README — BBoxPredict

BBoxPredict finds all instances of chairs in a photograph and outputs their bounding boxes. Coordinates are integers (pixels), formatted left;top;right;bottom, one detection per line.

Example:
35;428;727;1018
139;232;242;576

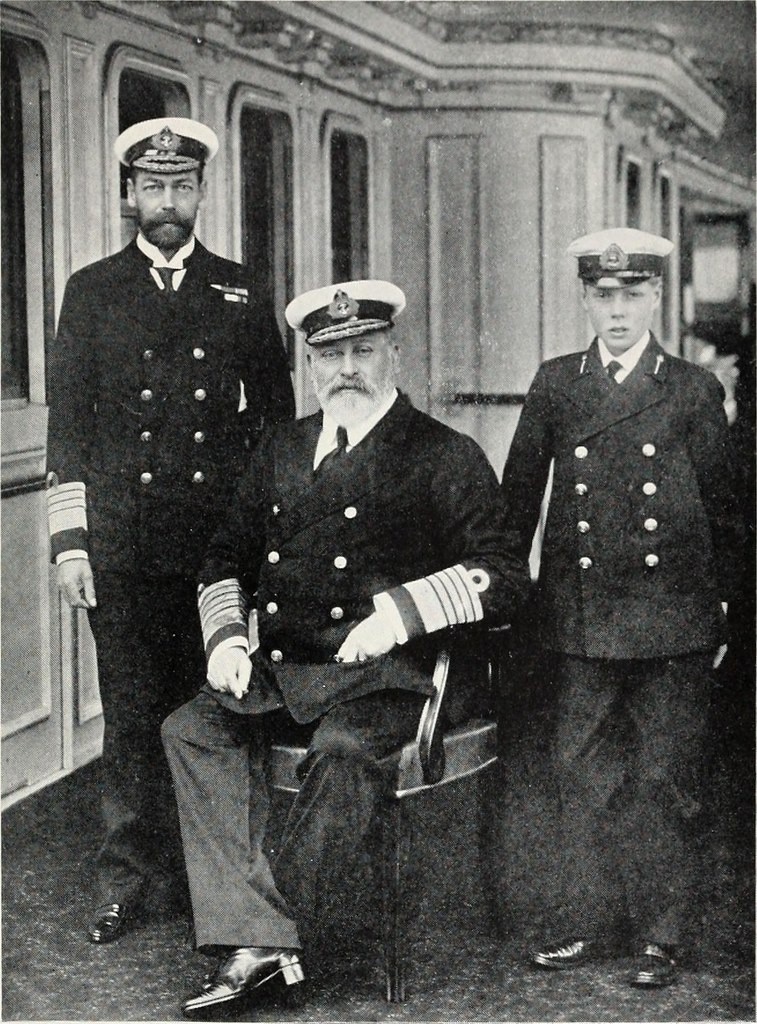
263;619;517;999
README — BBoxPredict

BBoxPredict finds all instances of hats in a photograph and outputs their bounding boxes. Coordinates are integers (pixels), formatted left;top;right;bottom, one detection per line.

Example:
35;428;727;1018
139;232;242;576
566;228;675;289
114;117;220;174
284;279;406;346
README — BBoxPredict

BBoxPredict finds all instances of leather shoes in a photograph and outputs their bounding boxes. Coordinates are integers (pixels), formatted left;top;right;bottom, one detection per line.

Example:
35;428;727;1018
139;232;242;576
180;946;305;1022
87;903;133;942
141;869;186;918
630;943;677;986
529;937;617;970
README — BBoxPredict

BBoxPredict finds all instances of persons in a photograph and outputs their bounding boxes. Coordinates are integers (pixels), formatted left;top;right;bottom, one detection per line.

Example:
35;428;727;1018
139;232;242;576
499;228;754;985
159;279;504;1014
46;117;296;945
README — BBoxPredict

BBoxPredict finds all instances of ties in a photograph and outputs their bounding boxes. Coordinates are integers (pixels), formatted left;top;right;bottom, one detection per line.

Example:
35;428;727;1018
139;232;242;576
150;266;185;299
606;361;623;382
312;427;348;482
